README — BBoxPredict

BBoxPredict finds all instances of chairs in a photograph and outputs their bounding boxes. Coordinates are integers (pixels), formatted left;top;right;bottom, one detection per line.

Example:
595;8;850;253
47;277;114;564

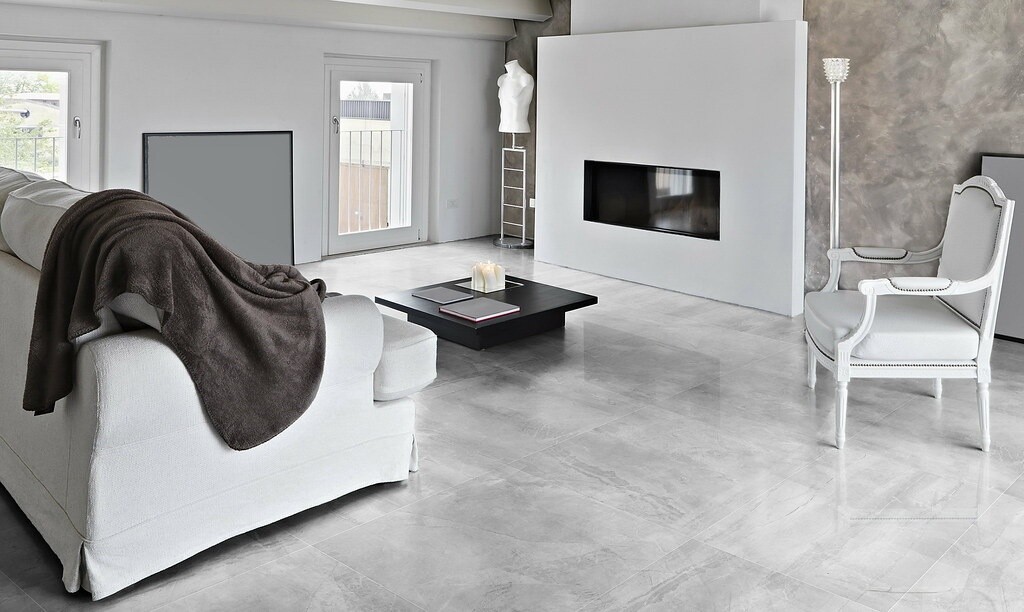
803;175;1015;453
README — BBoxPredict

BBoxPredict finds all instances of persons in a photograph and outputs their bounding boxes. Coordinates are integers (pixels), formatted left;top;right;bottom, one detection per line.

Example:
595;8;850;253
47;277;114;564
496;59;535;134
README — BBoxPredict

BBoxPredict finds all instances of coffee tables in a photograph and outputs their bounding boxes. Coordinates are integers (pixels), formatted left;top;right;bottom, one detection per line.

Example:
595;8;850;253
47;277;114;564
374;270;599;351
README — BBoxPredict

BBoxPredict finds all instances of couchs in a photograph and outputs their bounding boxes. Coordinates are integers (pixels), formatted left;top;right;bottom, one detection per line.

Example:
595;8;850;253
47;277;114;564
0;166;437;603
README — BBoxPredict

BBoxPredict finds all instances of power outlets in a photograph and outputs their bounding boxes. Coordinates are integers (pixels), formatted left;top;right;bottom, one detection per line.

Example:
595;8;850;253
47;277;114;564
529;198;535;208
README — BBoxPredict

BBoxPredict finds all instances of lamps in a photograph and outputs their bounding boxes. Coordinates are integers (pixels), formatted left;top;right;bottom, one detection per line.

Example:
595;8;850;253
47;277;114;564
823;58;851;290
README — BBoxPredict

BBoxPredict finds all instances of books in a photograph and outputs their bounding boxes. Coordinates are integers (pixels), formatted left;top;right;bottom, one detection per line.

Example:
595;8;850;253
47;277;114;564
439;297;520;323
411;286;474;305
